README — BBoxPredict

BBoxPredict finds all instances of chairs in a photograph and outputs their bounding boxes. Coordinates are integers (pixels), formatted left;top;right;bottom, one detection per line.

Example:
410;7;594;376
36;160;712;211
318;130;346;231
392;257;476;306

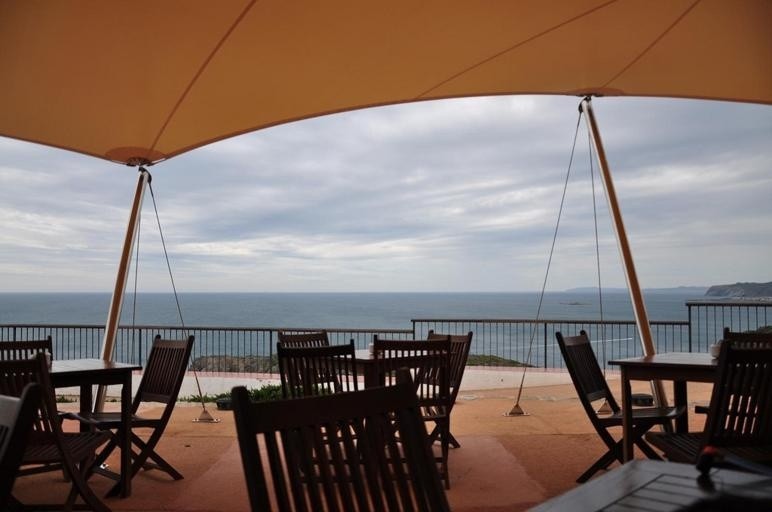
1;381;43;512
276;328;475;450
2;352;112;510
1;335;53;361
547;324;772;481
229;366;456;510
70;330;195;498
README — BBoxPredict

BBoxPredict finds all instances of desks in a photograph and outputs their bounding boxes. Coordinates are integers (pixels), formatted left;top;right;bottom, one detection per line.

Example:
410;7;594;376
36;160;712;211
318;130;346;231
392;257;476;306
3;357;144;496
527;460;772;510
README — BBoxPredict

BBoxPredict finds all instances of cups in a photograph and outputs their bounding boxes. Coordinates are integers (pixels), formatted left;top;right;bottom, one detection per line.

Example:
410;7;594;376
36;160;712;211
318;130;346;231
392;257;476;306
710;343;721;358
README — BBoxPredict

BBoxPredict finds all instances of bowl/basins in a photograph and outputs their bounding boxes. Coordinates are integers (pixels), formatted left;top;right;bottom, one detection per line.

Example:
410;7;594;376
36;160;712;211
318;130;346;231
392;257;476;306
29;352;51;370
368;343;383;354
